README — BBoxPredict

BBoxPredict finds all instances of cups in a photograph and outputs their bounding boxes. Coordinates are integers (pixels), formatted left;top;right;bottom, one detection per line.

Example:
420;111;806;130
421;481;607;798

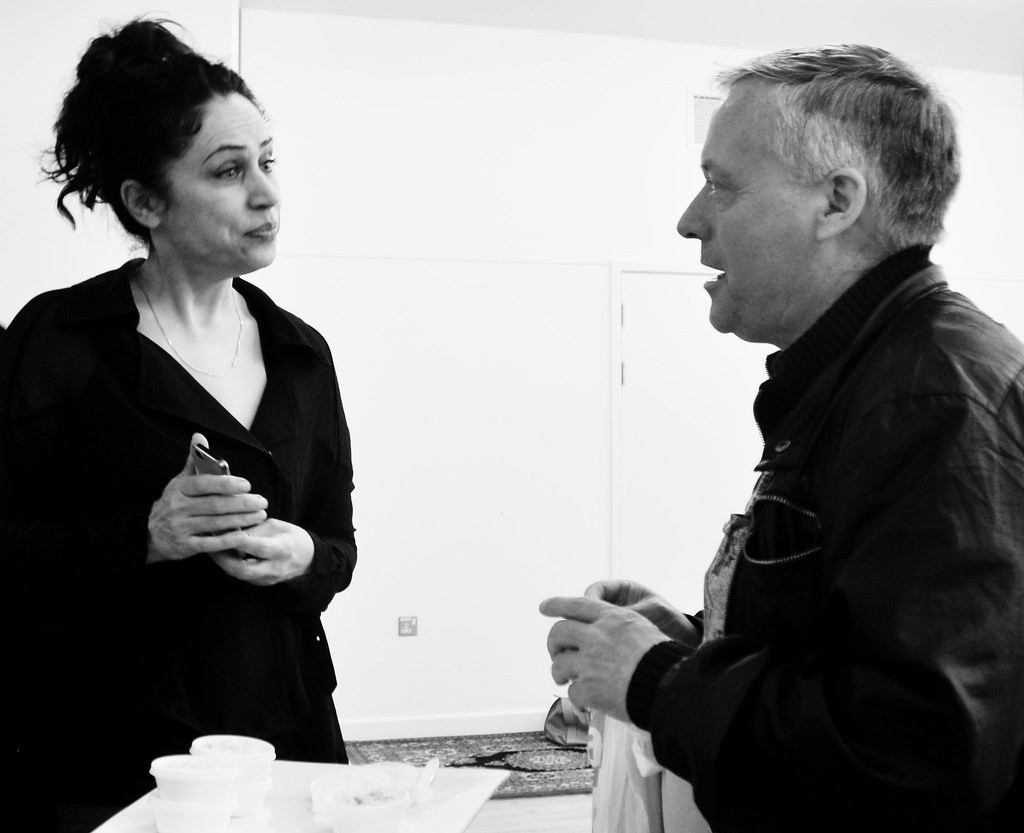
151;735;277;833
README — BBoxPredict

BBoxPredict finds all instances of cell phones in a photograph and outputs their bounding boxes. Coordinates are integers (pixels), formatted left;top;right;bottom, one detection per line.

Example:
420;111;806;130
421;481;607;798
192;443;247;559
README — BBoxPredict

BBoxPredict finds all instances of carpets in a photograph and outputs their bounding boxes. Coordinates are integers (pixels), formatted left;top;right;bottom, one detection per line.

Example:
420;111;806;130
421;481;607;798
343;731;591;799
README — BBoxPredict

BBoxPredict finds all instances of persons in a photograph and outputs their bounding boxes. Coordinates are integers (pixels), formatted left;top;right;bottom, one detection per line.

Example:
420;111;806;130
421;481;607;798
539;44;1022;833
0;18;359;833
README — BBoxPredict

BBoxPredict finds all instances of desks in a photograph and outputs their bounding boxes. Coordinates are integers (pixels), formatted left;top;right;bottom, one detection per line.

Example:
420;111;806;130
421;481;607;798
91;761;511;832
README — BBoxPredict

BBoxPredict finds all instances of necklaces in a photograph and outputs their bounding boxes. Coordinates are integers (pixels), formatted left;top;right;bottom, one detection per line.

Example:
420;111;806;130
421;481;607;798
136;266;243;376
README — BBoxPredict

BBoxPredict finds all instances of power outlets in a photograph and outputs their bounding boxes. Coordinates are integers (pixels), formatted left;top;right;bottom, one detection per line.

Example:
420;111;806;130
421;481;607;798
399;616;417;636
